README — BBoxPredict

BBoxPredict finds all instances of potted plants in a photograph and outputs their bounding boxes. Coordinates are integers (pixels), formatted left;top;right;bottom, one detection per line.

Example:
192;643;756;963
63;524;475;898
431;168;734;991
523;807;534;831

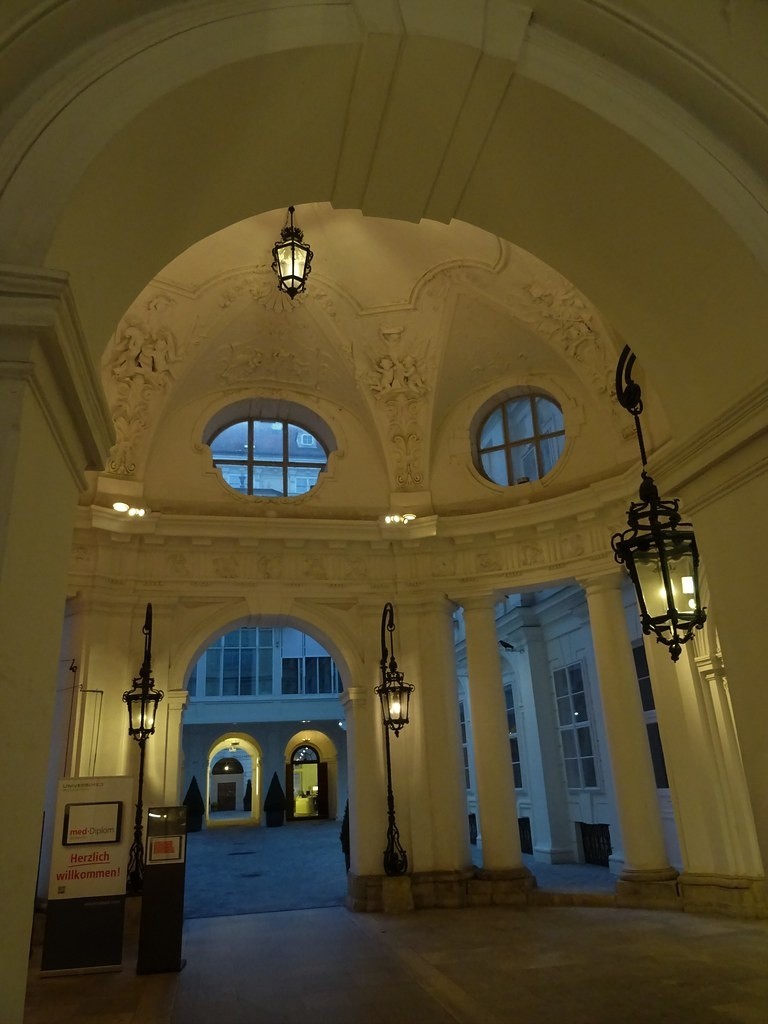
264;771;287;827
339;799;350;874
243;779;252;813
182;775;205;833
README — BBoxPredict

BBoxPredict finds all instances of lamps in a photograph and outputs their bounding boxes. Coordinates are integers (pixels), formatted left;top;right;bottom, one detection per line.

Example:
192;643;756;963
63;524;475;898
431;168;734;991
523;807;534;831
122;661;164;748
271;207;314;301
612;346;708;663
375;658;415;737
